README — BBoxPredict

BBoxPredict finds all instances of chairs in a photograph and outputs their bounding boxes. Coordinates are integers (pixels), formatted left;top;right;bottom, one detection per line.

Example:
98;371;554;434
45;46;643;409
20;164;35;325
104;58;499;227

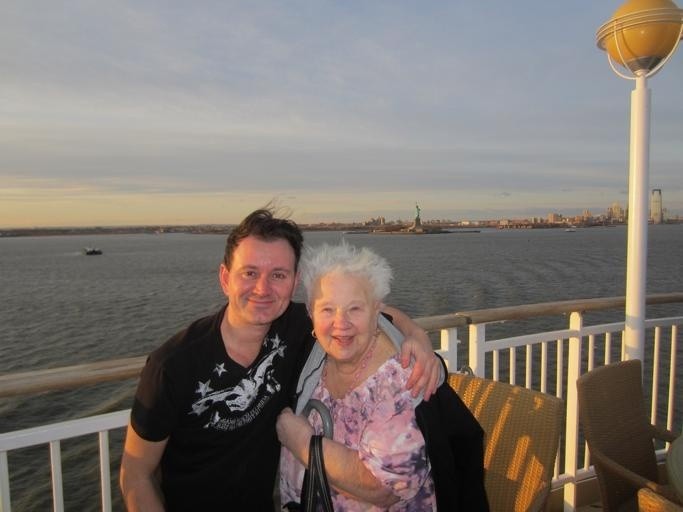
636;486;683;512
444;372;568;511
574;358;681;511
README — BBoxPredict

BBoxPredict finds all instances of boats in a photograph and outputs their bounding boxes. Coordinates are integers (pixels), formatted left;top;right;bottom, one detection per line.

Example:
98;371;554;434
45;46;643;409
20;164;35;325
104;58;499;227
565;226;575;232
82;247;105;255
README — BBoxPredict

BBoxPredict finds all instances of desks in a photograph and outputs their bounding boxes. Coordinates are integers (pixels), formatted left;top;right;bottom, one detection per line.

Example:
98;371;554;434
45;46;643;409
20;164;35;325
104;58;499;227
665;434;683;496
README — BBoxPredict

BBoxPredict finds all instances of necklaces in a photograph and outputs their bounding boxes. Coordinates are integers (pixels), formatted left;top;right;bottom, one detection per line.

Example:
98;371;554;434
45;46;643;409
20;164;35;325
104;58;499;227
320;338;377;401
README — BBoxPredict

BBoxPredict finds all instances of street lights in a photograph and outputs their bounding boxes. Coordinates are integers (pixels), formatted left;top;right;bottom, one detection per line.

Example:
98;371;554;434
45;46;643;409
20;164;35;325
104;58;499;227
589;1;683;383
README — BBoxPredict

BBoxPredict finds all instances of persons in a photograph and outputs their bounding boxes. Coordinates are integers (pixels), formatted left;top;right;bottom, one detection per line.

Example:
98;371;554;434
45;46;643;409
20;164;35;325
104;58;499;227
117;207;443;510
414;202;420;217
272;237;440;510
